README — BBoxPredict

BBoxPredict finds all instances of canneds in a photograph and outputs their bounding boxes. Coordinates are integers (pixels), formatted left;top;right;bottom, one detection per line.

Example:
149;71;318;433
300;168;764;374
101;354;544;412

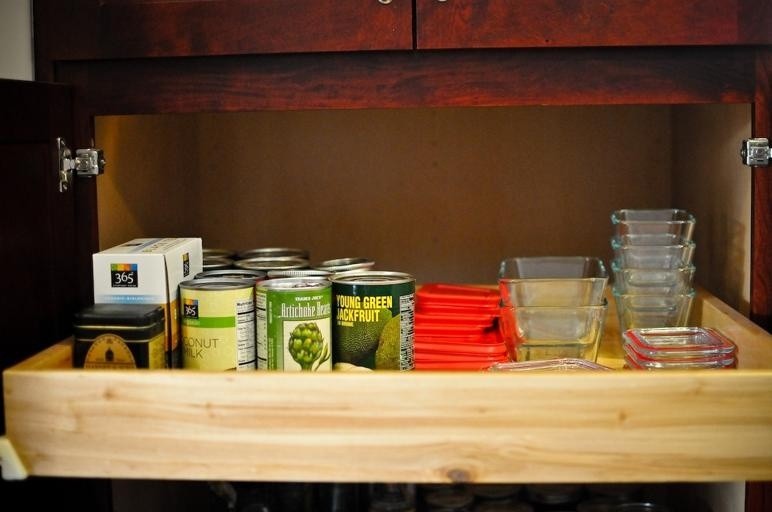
333;275;415;373
202;256;230;270
195;270;266;280
177;278;256;374
232;258;308;269
268;270;332;280
313;257;374;272
254;280;333;374
235;248;307;259
202;249;228;258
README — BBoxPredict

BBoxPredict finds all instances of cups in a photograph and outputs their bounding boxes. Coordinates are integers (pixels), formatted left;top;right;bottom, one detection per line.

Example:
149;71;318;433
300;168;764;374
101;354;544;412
496;208;738;369
